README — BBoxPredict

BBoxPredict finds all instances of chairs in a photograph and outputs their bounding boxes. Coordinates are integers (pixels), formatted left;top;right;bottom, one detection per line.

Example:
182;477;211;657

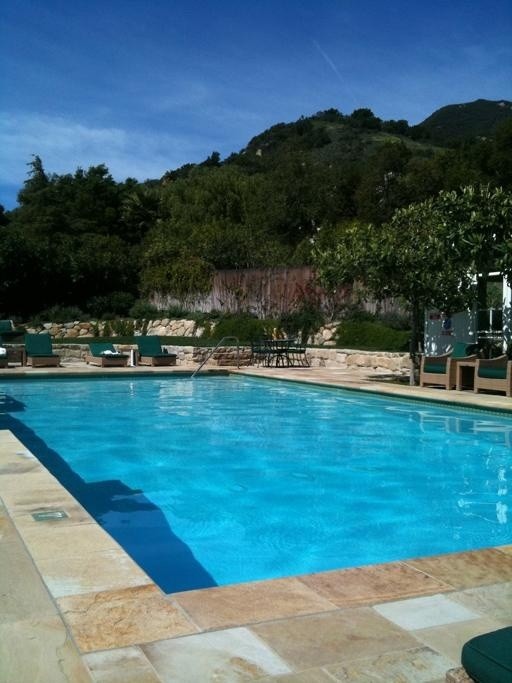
419;340;512;398
246;331;311;369
85;342;129;368
135;335;177;367
20;333;61;368
